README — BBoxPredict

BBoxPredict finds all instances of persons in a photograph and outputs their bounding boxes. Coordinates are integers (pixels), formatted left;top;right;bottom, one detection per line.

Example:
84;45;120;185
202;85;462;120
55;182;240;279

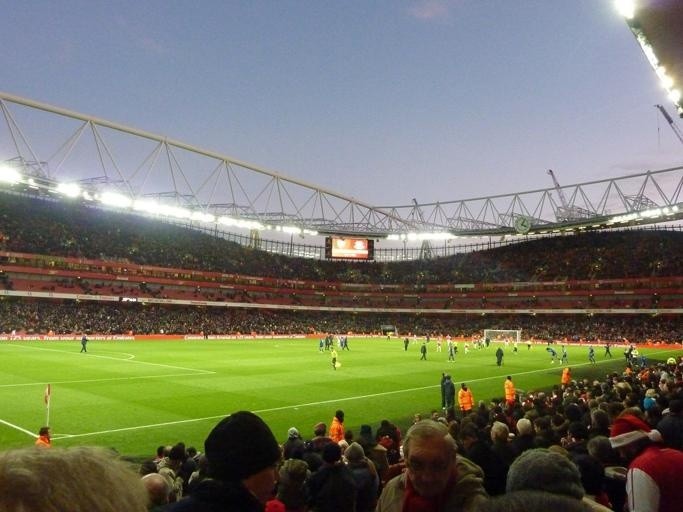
472;339;477;350
419;343;426;361
79;334;88;353
559;345;568;366
526;340;531;352
546;347;561;365
629;344;633;357
403;337;408;351
337;335;344;349
623;347;629;362
587;345;596;367
603;343;611;358
630;348;638;364
329;346;337;371
477;342;480;350
341;336;349;351
452;340;458;354
324;335;329;350
510;342;517;355
495;348;503;368
480;338;484;348
464;342;469;355
436;338;441;352
447;346;455;362
318;337;324;353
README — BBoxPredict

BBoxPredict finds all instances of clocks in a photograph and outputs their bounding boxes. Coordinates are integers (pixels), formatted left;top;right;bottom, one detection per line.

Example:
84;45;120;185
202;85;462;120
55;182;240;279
512;215;533;235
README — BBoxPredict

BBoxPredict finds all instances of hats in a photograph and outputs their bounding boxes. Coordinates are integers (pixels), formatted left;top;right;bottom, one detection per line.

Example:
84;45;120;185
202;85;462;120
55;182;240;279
313;422;326;432
202;410;278;482
609;414;661;449
287;427;298;437
345;442;365;460
666;357;677;365
